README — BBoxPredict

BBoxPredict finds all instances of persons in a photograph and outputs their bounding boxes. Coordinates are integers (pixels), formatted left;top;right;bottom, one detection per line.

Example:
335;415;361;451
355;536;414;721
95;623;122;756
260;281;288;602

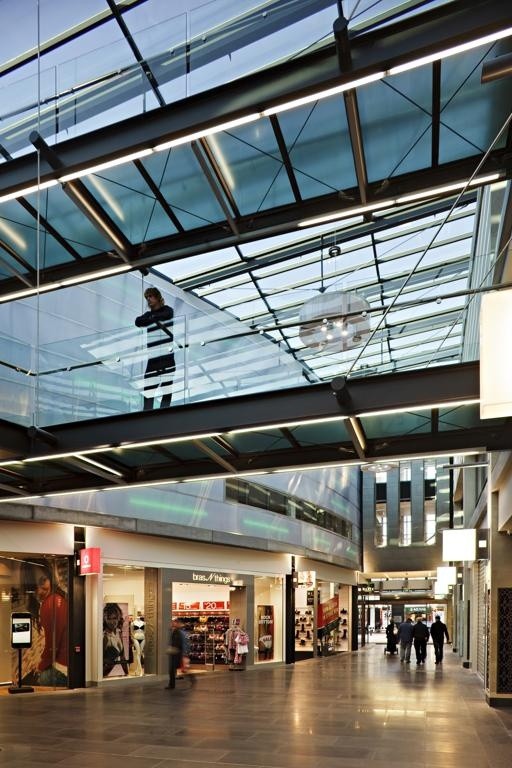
20;559;69;688
103;602;128;676
135;286;184;410
397;618;413;663
430;617;449;664
413;617;430;664
386;619;398;655
165;620;197;689
131;617;146;676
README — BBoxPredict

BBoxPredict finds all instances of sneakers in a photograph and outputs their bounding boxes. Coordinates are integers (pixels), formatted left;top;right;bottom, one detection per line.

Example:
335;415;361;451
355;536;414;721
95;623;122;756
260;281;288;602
164;684;175;689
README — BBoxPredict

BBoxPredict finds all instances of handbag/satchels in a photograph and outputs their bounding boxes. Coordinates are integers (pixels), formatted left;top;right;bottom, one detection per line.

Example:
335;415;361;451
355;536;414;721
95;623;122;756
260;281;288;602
165;646;180;655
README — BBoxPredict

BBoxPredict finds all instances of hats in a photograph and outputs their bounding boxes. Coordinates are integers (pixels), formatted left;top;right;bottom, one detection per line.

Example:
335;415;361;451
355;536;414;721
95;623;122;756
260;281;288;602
408;618;412;621
436;616;440;619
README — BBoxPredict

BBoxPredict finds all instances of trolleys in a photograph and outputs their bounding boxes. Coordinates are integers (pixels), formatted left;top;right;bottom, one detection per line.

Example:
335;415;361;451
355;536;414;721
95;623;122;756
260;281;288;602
383;636;398;655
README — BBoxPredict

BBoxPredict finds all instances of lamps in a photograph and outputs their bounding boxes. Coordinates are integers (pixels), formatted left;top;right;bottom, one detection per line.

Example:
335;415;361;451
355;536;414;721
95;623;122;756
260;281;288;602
298;222;372;352
